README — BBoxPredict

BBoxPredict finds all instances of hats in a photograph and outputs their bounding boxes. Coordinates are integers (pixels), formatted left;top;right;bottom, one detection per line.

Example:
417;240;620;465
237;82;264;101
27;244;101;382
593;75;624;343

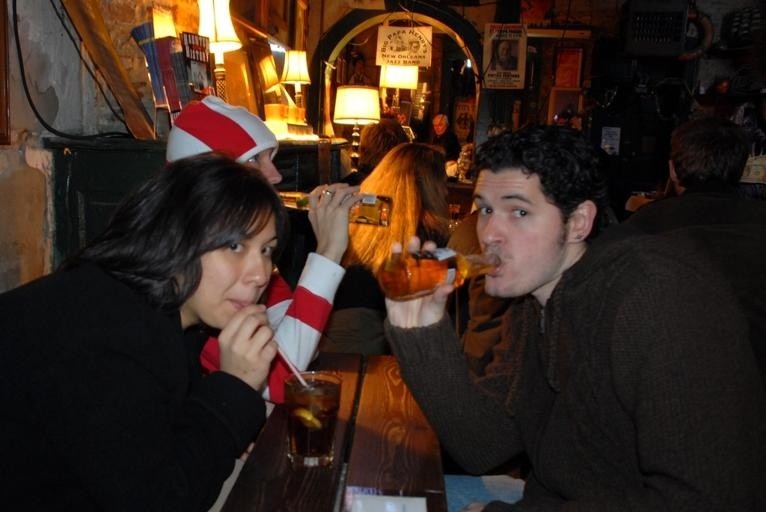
165;94;280;167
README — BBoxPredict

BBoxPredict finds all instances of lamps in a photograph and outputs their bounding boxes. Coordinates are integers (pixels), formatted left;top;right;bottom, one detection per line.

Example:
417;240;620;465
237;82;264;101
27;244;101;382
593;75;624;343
258;55;296;120
197;0;243;104
332;85;380;174
280;50;314;135
380;63;419;116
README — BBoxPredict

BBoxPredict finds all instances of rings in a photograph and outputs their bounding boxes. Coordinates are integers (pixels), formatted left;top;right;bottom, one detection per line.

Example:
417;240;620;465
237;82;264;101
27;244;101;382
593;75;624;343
322;191;334;196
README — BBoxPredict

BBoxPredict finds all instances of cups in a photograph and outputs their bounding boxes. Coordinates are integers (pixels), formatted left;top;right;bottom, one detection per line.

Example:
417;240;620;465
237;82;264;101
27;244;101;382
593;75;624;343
448;203;459;229
283;371;340;469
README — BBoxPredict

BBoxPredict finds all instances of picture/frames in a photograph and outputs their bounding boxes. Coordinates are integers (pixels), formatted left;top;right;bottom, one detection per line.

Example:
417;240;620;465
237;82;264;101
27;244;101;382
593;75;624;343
552;46;583;88
547;87;585;129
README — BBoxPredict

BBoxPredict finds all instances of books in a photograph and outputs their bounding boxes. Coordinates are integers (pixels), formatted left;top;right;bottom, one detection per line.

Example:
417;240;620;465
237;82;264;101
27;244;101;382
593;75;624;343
130;20;213;128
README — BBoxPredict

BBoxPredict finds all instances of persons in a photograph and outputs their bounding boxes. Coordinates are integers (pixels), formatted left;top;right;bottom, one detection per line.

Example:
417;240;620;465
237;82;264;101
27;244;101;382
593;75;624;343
492;42;518;72
446;210;524;379
429;114;461;164
162;93;368;409
616;115;766;366
0;147;292;512
278;142;469;361
377;125;766;510
337;116;412;192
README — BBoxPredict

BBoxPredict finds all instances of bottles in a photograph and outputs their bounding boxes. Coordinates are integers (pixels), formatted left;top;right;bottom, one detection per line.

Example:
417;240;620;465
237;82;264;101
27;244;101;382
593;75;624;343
273;190;394;226
377;251;502;302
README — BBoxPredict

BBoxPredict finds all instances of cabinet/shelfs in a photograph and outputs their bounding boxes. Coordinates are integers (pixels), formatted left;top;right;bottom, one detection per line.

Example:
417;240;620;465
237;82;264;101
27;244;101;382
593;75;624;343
46;138;340;280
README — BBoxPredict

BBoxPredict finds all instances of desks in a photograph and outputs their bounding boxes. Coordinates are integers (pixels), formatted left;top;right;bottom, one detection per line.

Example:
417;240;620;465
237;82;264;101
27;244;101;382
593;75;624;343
220;352;445;512
